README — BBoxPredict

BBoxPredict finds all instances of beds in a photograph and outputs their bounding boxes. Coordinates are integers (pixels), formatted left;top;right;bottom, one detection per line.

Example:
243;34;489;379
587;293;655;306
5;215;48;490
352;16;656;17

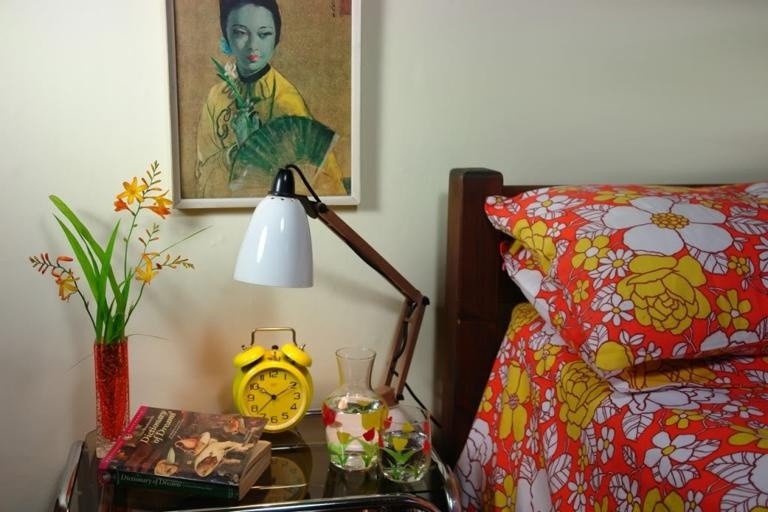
438;163;768;511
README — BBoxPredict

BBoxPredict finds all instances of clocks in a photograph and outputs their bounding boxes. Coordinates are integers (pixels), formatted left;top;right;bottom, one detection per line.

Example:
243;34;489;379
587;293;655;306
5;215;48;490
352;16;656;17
230;325;316;436
247;451;311;506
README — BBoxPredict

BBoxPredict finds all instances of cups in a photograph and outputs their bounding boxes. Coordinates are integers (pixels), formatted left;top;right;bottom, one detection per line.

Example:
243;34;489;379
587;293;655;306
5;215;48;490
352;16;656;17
379;405;431;482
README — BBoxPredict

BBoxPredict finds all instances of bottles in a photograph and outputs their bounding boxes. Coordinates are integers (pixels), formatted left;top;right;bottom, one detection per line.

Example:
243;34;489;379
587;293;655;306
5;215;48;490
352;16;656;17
321;346;389;473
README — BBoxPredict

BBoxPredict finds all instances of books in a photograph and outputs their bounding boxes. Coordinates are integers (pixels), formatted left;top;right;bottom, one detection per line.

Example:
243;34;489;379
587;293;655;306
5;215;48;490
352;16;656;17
97;404;272;502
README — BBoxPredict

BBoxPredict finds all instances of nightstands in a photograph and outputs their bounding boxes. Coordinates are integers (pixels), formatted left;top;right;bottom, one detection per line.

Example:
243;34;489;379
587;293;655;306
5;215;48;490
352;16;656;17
53;408;462;512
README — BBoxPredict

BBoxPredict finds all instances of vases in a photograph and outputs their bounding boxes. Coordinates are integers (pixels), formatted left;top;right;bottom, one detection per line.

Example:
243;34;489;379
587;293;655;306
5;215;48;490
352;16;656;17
92;335;131;445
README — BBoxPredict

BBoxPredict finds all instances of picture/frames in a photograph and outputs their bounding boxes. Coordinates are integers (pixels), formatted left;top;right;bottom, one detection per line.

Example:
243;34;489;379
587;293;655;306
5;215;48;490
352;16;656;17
165;0;361;212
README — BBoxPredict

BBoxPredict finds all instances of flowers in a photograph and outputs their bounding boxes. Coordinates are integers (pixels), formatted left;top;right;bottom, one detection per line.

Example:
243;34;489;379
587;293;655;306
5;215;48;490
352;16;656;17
24;158;216;349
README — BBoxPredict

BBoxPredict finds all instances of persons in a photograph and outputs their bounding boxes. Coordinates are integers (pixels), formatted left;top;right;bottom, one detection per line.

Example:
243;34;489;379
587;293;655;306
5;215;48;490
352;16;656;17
195;0;347;199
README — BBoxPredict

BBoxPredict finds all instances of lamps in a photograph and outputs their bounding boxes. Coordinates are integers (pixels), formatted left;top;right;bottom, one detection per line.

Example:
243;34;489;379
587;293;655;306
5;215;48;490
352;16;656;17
231;161;432;408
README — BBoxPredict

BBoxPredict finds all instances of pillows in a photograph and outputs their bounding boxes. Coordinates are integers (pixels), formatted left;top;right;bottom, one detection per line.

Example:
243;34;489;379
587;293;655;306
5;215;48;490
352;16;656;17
481;179;768;394
